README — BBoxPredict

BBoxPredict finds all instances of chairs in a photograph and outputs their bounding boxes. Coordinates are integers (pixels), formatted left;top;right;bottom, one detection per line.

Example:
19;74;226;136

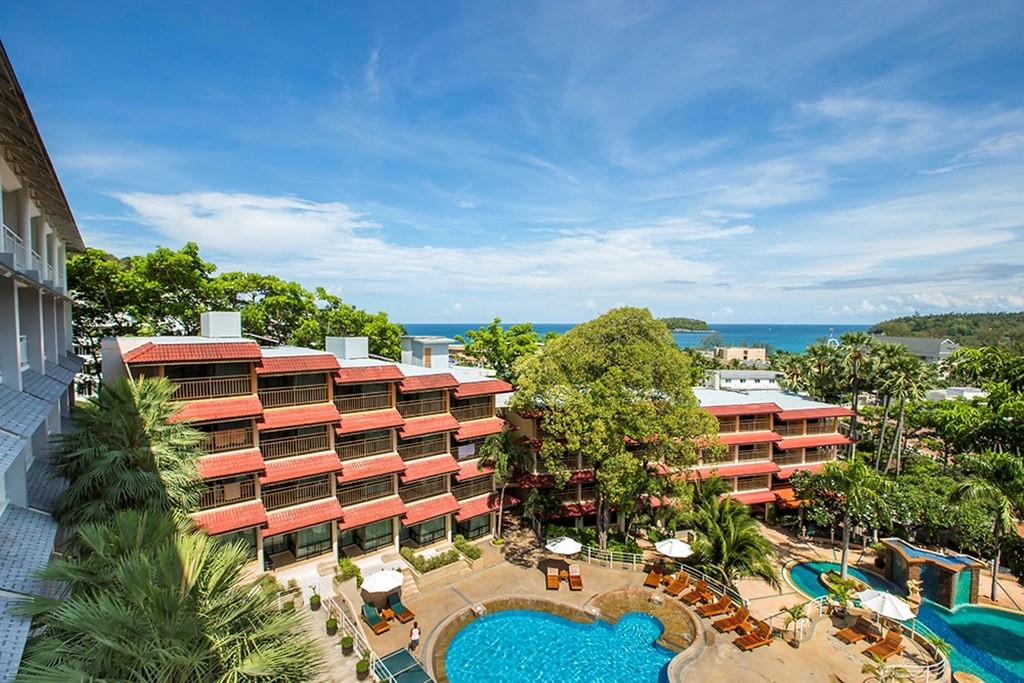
836;616;873;645
682;579;708;604
735;620;776;652
546;564;560;589
696;595;735;620
664;571;691;596
714;606;751;632
387;592;415;624
642;561;665;588
361;604;390;636
866;630;906;663
568;564;583;590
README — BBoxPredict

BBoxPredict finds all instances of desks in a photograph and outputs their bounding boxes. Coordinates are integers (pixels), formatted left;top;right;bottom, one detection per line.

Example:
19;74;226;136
701;592;714;605
737;623;753;636
560;570;568;581
381;608;396;623
662;575;674;586
866;631;882;645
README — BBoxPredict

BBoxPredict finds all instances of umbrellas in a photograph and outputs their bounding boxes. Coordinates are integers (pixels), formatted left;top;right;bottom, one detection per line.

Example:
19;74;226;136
545;536;583;561
359;570;405;595
654;539;693;570
856;588;917;634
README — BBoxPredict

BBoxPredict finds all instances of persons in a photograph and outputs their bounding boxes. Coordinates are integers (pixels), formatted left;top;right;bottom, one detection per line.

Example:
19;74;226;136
410;622;421;654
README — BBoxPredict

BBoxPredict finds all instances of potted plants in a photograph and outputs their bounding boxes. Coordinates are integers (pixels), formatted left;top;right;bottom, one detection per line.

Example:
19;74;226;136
779;603;814;649
308;586;371;680
922;632;954;671
331;557;361;595
833;584;848;618
